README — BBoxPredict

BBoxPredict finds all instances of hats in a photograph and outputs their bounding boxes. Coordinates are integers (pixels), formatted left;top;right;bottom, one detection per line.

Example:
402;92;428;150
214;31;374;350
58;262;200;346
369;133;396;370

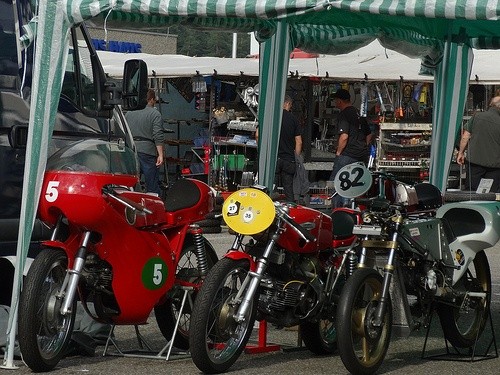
330;89;350;100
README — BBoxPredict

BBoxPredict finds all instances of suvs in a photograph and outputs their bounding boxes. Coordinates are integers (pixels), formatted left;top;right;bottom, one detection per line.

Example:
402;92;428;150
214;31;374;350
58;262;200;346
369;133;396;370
0;0;148;259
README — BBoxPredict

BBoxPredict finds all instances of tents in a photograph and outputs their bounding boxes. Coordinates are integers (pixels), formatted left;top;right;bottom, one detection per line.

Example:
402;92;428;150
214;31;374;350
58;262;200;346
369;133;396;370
0;0;500;369
67;40;500;109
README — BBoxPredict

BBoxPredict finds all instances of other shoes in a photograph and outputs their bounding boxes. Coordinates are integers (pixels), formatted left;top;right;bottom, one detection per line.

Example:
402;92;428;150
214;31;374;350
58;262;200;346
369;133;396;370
89;327;116;346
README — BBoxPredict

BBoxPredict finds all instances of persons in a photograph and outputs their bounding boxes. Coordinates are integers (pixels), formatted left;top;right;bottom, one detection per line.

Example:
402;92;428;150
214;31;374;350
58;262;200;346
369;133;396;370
122;90;165;193
457;95;500;197
329;88;372;215
254;94;303;205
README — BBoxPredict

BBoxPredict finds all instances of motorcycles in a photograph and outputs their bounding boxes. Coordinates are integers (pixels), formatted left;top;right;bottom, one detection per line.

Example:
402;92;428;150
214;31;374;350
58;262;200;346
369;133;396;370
188;171;364;374
327;172;500;375
17;137;219;372
